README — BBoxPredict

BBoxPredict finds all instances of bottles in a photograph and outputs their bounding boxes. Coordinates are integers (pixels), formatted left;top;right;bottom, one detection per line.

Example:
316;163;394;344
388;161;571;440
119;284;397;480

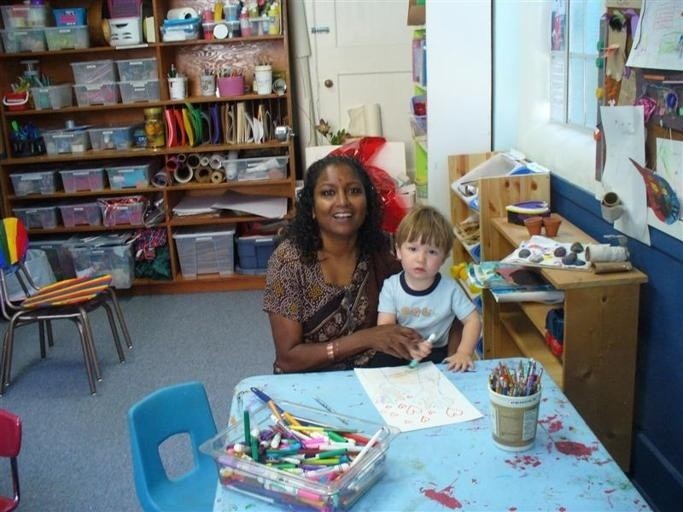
202;0;279;40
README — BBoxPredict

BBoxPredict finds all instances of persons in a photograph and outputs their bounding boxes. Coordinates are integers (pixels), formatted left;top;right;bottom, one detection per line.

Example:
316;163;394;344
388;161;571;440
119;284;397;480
262;154;422;374
377;206;482;373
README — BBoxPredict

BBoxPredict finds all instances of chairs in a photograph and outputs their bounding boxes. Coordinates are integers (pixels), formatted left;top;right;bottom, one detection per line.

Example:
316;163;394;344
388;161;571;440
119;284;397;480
0;412;21;512
1;218;132;396
127;379;220;511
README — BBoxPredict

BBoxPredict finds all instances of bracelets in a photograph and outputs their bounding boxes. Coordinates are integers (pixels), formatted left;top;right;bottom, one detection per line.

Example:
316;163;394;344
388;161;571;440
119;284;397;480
326;341;334;364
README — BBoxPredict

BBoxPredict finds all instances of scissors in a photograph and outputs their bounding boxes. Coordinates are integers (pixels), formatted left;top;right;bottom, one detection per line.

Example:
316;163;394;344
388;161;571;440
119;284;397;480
12;121;44;155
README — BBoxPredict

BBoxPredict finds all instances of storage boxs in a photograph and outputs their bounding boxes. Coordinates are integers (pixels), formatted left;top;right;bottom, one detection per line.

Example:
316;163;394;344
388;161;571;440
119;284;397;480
233;233;280;277
171;225;236;278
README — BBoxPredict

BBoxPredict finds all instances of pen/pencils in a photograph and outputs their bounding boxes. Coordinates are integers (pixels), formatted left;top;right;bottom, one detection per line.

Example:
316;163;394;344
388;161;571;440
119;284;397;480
201;65;244;77
32;73;52;87
166;63;180;78
488;357;544;397
219;387;384;512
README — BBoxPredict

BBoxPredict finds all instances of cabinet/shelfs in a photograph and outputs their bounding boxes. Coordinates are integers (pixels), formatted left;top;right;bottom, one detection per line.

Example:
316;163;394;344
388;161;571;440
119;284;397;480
41;120;134;155
1;1;89;52
447;151;551;361
30;57;162;112
409;24;427;155
6;163;153;197
0;1;299;296
488;213;648;474
28;235;136;294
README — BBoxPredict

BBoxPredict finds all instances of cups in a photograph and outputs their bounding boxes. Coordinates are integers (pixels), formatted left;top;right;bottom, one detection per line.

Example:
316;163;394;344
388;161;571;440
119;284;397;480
523;216;543;236
218;75;245;97
168;77;188;99
487;378;544;452
543;216;562;237
254;65;273;95
200;75;216;96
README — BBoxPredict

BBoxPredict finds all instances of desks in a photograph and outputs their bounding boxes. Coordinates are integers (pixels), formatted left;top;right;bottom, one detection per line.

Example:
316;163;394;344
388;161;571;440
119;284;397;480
211;357;650;511
